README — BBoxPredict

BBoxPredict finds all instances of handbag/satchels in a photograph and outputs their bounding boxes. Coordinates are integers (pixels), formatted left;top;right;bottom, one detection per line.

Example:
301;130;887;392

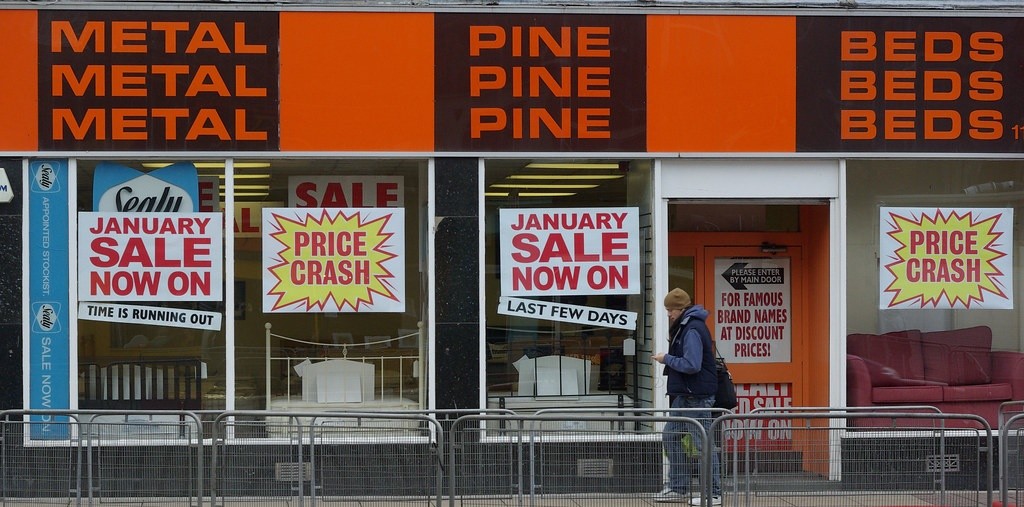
713;370;737;413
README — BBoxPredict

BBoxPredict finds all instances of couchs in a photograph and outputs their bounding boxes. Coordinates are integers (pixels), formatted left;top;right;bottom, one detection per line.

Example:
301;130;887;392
846;326;1023;429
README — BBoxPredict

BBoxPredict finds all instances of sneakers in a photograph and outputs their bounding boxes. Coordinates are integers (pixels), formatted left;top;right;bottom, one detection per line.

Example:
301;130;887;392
688;495;722;505
654;489;688;502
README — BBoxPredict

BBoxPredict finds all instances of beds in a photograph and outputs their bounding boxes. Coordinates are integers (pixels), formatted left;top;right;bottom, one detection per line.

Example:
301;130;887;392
485;325;640;436
78;357;203;439
265;322;425;438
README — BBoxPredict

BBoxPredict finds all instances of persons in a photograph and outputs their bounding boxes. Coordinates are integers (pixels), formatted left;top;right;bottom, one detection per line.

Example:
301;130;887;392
651;287;723;506
522;326;565;358
578;324;602;354
120;337;175;367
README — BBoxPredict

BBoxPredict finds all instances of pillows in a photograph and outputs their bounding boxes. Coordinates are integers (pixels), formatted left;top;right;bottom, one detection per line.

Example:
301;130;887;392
847;326;992;385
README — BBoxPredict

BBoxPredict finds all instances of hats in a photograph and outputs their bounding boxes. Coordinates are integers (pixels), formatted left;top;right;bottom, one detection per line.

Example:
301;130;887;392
664;288;691;309
